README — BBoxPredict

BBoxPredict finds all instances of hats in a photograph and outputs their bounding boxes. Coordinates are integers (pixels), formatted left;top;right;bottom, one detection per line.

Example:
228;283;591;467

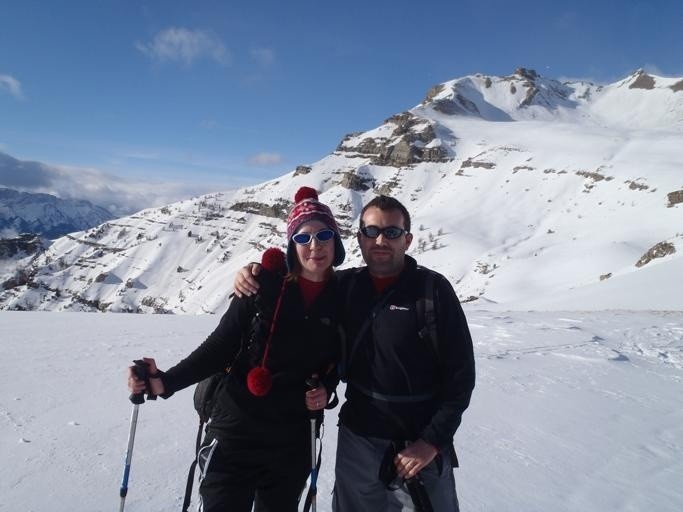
286;187;344;274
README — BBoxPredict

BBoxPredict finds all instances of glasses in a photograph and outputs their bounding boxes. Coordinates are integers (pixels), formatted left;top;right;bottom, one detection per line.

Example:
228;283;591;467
292;230;334;245
361;225;407;240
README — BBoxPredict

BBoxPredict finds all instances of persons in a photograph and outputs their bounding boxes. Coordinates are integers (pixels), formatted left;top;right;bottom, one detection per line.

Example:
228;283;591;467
234;197;477;512
123;186;346;512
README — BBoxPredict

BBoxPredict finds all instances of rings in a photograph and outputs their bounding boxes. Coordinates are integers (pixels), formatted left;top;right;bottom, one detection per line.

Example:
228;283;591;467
406;463;412;470
316;401;319;406
